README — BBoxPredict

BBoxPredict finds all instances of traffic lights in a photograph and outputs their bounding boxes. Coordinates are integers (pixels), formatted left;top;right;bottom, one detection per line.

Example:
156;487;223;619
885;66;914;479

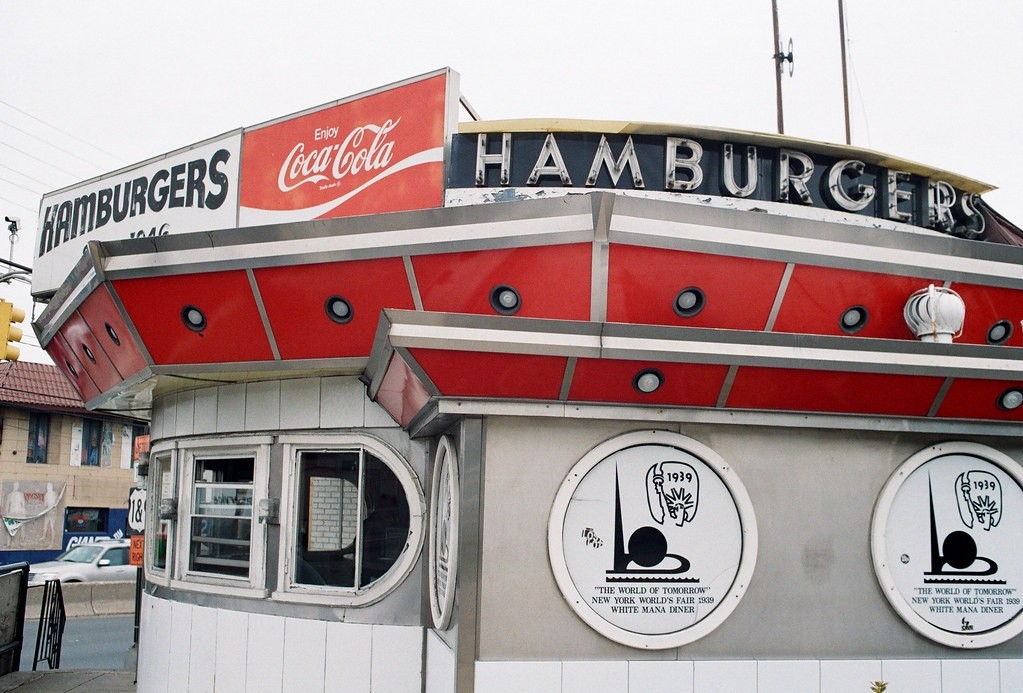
0;298;26;362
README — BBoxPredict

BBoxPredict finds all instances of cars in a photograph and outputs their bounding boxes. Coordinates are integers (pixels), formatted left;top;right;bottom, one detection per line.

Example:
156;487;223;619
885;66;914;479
27;538;138;588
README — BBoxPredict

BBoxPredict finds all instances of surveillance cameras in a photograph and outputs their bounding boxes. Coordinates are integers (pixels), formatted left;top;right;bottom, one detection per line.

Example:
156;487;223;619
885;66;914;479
5;216;21;222
